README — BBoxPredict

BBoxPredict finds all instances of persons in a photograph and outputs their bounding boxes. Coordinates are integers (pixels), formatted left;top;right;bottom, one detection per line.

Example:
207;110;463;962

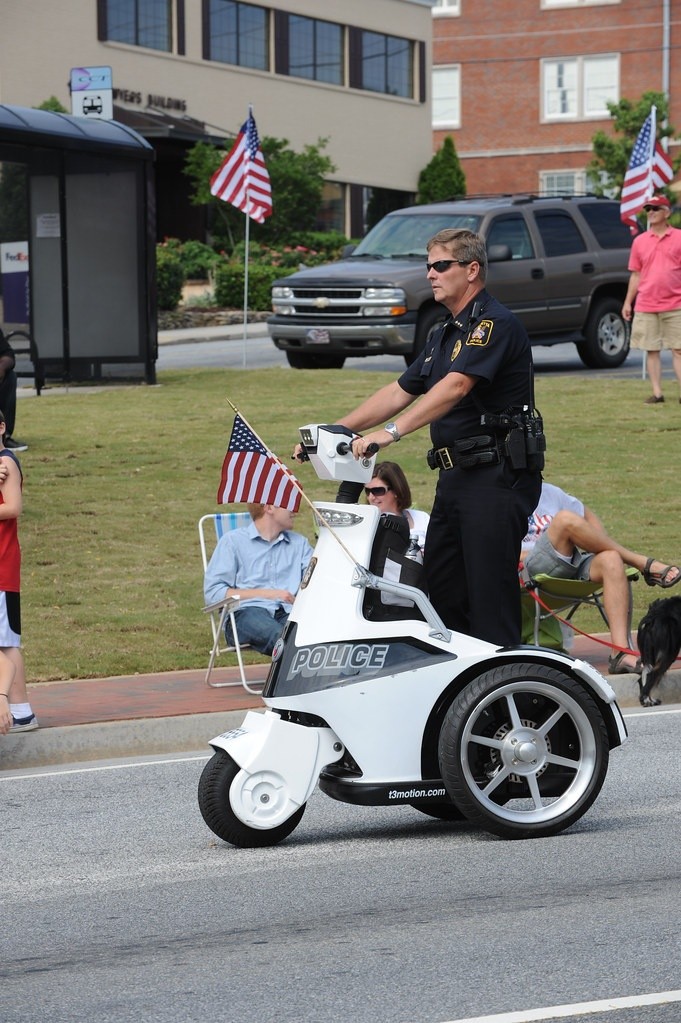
294;228;543;650
0;409;40;734
621;196;681;406
518;481;681;674
0;317;29;453
203;501;316;656
366;461;431;564
0;647;14;734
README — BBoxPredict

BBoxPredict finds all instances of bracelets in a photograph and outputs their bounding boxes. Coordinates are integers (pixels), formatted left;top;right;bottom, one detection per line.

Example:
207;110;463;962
0;693;8;698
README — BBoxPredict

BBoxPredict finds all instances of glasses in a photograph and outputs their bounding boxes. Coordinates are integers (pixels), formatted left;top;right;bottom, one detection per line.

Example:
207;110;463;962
646;206;664;212
427;260;466;272
365;487;393;496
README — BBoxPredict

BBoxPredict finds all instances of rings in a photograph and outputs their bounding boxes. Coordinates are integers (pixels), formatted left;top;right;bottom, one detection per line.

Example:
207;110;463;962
361;438;365;443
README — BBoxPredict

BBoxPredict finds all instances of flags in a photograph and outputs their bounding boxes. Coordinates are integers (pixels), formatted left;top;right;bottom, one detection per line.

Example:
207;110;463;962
210;107;272;223
618;112;674;234
216;416;303;515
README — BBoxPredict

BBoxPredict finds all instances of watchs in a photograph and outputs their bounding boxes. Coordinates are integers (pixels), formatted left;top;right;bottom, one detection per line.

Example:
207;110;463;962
385;423;400;443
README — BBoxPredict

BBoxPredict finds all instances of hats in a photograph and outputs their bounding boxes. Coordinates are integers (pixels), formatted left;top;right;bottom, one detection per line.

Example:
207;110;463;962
643;193;670;208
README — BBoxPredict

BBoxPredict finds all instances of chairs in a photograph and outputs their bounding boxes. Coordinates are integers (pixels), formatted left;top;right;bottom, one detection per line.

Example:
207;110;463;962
518;566;641;651
197;511;264;695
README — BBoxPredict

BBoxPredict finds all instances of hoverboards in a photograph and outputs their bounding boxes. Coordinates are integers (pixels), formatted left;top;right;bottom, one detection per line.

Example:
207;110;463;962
195;421;630;850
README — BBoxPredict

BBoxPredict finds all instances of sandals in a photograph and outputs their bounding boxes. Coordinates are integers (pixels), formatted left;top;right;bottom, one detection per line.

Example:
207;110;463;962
609;651;643;674
642;558;681;588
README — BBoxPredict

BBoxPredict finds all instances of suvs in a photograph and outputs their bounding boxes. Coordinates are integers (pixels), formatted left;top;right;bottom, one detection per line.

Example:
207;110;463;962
263;187;641;371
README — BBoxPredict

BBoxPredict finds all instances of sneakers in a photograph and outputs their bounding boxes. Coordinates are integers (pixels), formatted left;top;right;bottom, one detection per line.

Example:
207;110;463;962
8;714;39;734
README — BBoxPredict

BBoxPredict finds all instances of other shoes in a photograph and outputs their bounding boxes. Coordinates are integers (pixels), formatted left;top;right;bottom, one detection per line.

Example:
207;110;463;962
3;438;27;451
647;396;664;403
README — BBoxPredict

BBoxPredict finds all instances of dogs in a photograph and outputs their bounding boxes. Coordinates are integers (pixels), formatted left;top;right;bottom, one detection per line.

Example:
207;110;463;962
638;595;681;708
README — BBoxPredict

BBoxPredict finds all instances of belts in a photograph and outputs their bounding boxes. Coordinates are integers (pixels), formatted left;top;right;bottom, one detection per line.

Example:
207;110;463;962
434;447;468;471
